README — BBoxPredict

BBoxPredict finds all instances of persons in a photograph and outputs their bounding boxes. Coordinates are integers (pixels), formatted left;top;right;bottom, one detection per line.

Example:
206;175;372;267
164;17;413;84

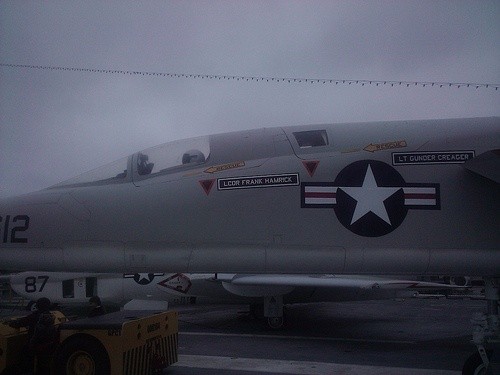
88;296;105;317
181;148;205;164
5;296;50;351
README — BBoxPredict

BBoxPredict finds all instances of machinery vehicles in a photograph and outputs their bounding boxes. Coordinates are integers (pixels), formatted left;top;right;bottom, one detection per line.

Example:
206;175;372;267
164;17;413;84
0;307;180;375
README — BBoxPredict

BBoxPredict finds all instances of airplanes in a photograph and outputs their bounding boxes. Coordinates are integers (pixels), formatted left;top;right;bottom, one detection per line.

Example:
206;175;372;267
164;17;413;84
0;115;500;375
